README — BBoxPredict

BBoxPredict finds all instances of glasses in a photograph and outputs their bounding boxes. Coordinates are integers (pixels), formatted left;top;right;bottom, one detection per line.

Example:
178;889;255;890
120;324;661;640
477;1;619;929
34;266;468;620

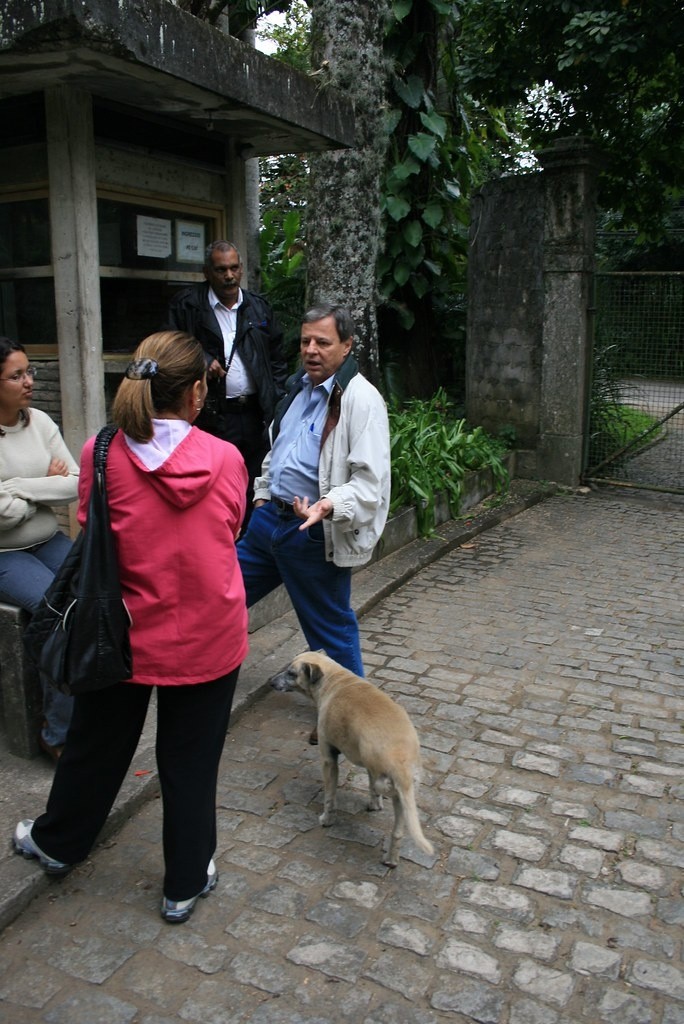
0;365;37;383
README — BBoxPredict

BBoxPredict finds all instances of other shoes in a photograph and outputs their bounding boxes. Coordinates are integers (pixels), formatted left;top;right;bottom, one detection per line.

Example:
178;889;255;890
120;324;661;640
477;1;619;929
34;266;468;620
39;736;66;762
309;727;319;744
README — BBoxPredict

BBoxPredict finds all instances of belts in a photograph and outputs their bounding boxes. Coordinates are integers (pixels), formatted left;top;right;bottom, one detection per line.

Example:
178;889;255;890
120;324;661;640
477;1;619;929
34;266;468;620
229;394;258;404
270;495;295;512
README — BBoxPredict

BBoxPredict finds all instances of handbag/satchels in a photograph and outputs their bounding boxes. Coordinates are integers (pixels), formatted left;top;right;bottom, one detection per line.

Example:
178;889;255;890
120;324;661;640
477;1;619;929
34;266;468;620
30;425;125;696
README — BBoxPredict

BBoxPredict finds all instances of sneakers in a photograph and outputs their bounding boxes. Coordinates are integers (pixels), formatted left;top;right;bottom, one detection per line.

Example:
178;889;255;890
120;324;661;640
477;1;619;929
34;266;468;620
161;859;219;923
12;818;71;873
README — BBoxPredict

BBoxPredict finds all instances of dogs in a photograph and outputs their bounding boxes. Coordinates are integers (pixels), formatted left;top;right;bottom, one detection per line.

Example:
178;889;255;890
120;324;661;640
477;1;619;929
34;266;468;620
269;648;434;868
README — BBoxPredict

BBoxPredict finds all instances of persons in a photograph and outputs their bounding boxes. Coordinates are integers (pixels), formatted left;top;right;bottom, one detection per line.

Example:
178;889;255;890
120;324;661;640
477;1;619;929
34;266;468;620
236;306;391;744
0;337;81;763
169;239;289;543
14;330;249;924
